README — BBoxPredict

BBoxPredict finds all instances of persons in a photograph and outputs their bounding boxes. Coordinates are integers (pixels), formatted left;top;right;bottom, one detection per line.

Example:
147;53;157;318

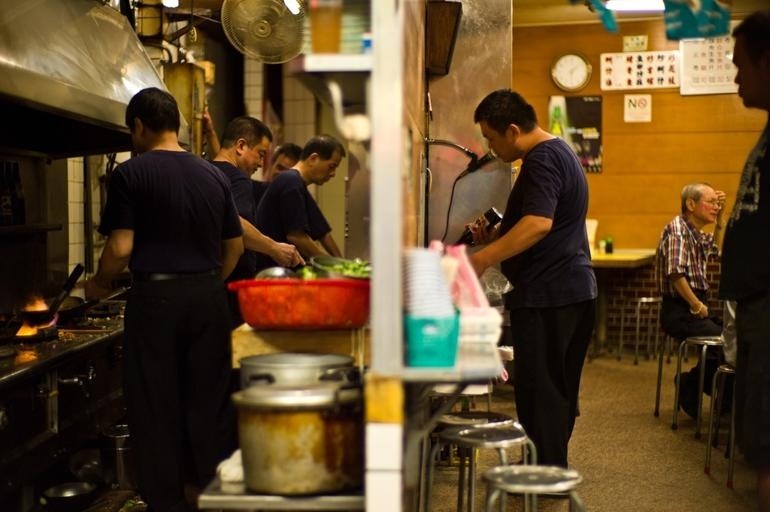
706;10;770;512
658;183;727;421
93;88;244;512
209;117;306;329
254;134;346;269
466;89;595;498
250;143;303;206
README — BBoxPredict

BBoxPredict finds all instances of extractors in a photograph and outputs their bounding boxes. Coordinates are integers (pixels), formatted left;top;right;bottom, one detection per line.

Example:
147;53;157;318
1;0;194;155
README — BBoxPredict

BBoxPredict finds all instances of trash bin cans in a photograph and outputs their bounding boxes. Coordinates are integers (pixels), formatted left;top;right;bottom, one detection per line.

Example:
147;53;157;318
101;425;132;482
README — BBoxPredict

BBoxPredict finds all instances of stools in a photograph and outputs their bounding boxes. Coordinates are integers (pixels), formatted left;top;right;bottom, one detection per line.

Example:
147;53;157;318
419;379;587;512
673;335;723;440
704;362;741;489
654;330;684;417
617;292;663;366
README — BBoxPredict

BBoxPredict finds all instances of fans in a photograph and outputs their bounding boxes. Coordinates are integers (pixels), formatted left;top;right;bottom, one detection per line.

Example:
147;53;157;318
221;0;311;66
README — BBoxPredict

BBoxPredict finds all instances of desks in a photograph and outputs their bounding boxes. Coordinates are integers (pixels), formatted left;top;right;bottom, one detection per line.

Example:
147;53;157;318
198;469;367;512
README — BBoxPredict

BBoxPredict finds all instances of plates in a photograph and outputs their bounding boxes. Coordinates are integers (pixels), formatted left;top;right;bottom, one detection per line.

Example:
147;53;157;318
297;13;368;56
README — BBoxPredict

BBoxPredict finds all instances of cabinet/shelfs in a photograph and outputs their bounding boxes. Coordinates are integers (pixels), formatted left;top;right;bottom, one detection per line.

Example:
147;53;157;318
402;360;505;454
286;50;370;143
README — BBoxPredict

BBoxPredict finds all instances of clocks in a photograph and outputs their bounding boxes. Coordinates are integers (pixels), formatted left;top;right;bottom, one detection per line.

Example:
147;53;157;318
549;48;593;94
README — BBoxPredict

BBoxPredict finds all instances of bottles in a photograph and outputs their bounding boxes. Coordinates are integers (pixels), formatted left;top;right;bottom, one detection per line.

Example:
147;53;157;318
451;205;504;247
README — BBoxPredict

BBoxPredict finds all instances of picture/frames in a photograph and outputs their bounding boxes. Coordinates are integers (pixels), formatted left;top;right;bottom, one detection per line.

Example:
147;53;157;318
679;37;739;96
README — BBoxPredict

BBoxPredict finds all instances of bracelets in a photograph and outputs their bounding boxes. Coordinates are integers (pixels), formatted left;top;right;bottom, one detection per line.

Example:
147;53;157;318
689;301;704;315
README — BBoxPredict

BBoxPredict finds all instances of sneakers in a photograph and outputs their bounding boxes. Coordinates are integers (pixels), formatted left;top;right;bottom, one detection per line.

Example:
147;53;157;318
674;368;713;417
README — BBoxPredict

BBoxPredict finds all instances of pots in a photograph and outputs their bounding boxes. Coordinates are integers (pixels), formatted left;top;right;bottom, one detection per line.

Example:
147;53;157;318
229;352;364;499
0;264;131;350
